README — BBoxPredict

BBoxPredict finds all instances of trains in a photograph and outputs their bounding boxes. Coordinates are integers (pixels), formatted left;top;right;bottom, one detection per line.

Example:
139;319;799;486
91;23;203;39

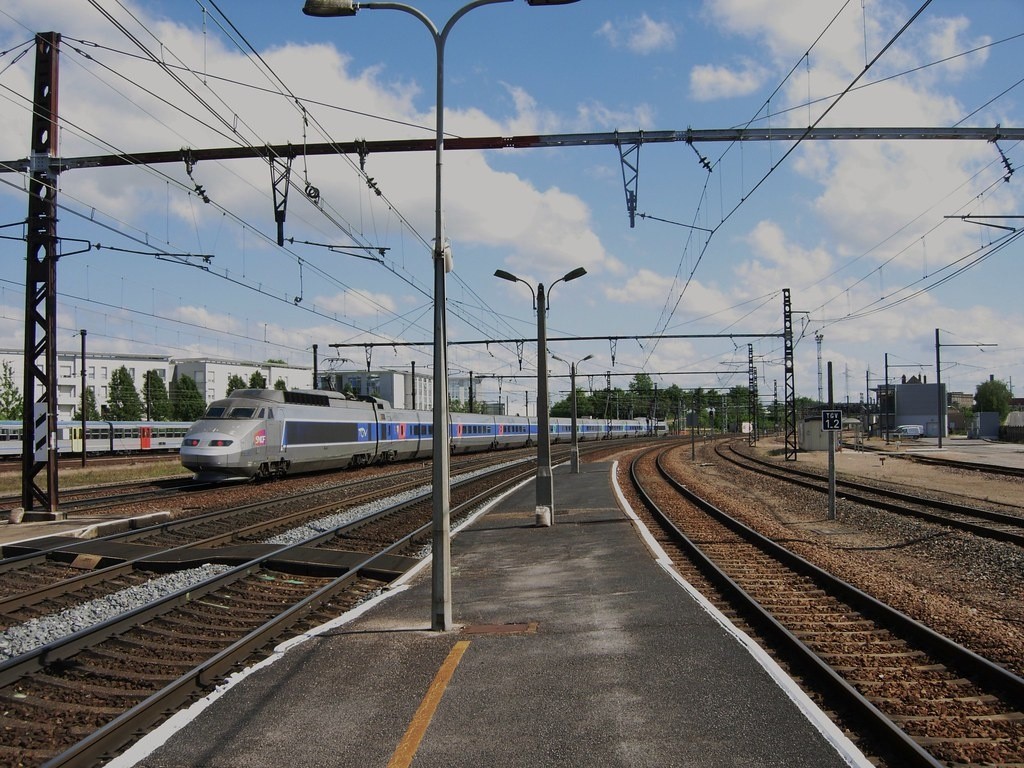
179;388;669;485
0;419;194;459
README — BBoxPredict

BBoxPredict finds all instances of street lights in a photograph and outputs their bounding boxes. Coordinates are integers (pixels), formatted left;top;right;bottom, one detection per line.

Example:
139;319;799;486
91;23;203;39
551;353;594;473
298;0;581;637
492;267;586;528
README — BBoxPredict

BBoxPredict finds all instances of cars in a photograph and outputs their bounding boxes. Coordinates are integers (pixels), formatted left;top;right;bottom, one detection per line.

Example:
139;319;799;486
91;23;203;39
896;425;924;438
